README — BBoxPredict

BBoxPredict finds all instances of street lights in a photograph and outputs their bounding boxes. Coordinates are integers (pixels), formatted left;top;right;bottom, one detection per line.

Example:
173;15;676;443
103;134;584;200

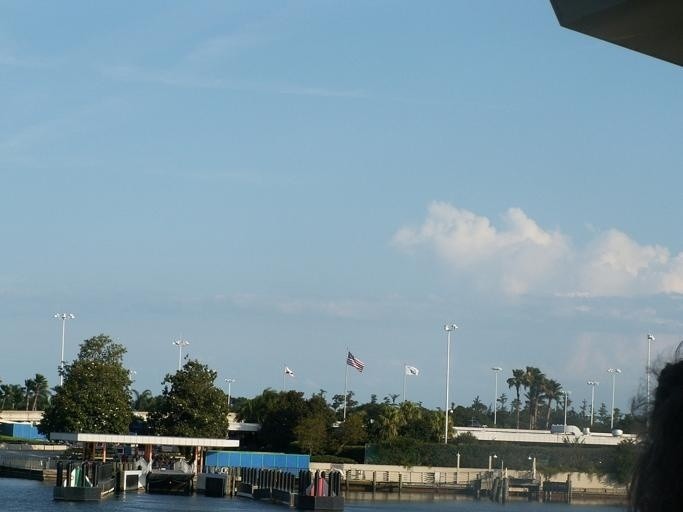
174;341;189;376
54;313;75;387
443;325;459;447
224;378;235;415
587;381;600;428
128;371;137;406
646;334;655;432
608;368;622;431
492;367;503;427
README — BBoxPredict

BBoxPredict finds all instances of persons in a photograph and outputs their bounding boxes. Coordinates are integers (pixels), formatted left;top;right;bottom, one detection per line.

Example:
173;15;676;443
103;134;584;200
625;340;683;512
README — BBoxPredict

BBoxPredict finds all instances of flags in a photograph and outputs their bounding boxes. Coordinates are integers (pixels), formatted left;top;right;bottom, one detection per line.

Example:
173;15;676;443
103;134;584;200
404;364;419;376
284;366;293;377
346;350;363;373
